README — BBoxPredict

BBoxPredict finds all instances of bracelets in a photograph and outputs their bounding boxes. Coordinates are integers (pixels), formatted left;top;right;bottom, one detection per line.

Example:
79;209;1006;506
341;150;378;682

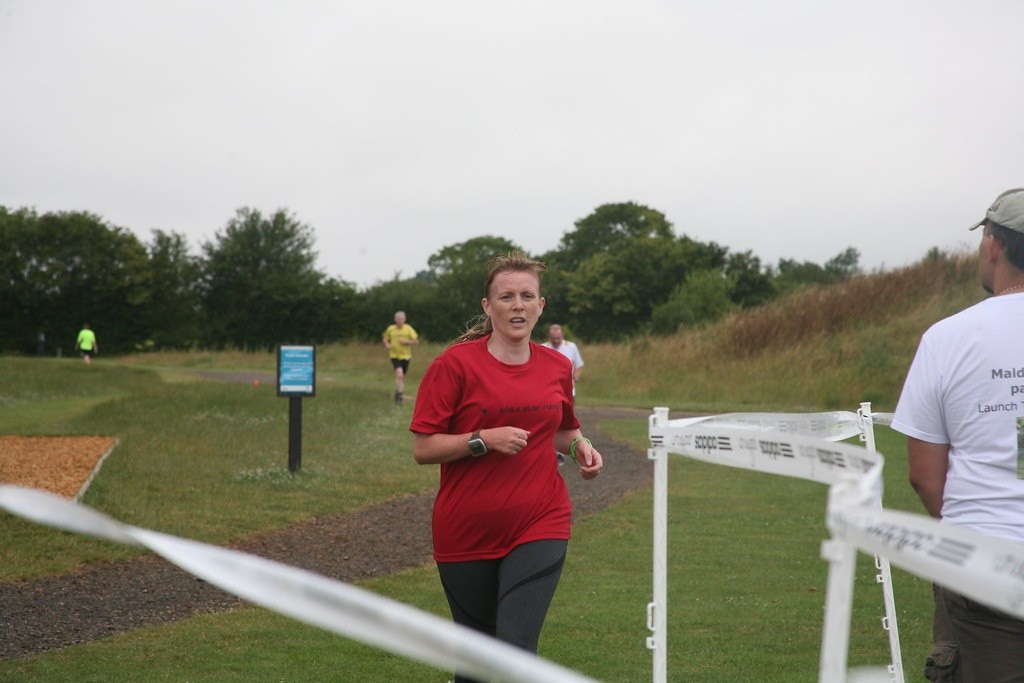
573;376;577;383
570;437;592;464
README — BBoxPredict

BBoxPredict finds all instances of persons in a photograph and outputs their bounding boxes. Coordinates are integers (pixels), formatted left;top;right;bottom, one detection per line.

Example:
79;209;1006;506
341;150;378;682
74;323;98;364
890;186;1024;683
410;250;604;683
382;311;420;405
540;324;584;396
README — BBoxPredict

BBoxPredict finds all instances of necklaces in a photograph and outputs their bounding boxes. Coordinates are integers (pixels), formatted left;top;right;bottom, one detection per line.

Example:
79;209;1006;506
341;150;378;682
999;284;1024;295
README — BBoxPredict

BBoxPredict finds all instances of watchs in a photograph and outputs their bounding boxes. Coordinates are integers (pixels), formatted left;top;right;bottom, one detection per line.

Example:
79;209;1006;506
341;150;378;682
468;430;489;457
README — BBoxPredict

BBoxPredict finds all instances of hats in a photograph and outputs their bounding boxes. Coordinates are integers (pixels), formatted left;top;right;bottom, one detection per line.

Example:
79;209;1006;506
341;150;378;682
968;186;1024;234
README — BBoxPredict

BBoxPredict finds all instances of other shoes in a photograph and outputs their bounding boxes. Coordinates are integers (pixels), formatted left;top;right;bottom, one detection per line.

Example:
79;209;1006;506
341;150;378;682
395;390;405;400
557;452;565;467
